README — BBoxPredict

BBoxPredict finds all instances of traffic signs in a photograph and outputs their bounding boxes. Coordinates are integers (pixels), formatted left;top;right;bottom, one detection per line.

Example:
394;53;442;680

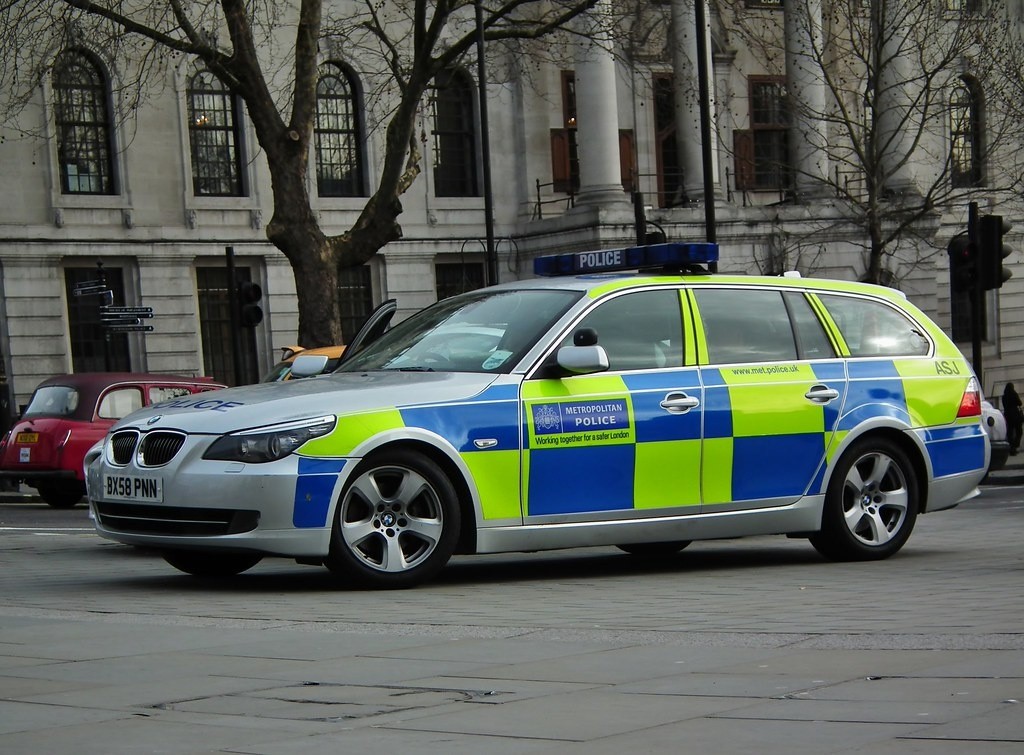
73;280;154;332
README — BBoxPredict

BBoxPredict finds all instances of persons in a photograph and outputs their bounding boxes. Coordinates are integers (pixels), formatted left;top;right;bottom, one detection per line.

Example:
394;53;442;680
1000;383;1022;456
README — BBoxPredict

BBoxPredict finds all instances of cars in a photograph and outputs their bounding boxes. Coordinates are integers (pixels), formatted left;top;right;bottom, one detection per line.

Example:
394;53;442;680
0;372;230;509
84;242;1009;591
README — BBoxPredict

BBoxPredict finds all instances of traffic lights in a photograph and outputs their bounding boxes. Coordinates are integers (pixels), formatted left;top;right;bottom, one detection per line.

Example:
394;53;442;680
237;281;263;328
947;235;983;301
979;214;1012;291
